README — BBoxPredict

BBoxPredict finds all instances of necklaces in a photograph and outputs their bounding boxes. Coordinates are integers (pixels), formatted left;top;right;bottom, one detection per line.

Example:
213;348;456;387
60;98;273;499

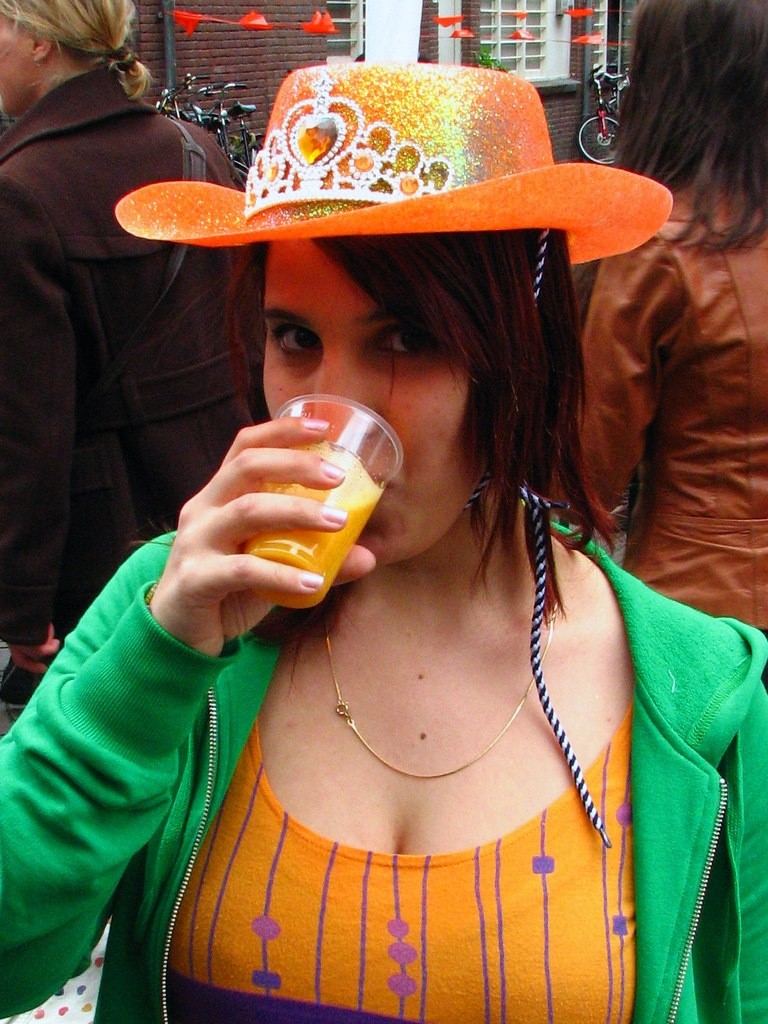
324;582;558;778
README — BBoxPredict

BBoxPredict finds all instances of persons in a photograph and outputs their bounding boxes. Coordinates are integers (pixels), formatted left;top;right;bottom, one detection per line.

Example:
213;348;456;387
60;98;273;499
0;0;768;1024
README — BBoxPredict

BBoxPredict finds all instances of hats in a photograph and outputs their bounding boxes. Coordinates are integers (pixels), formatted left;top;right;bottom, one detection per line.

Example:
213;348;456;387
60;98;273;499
116;61;673;266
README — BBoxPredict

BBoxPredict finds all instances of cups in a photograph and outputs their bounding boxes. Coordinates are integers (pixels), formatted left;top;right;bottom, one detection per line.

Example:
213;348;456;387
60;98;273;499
242;393;404;610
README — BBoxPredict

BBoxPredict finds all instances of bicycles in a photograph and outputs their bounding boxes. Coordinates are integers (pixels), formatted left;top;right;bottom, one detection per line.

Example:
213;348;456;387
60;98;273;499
154;72;266;188
577;64;631;166
